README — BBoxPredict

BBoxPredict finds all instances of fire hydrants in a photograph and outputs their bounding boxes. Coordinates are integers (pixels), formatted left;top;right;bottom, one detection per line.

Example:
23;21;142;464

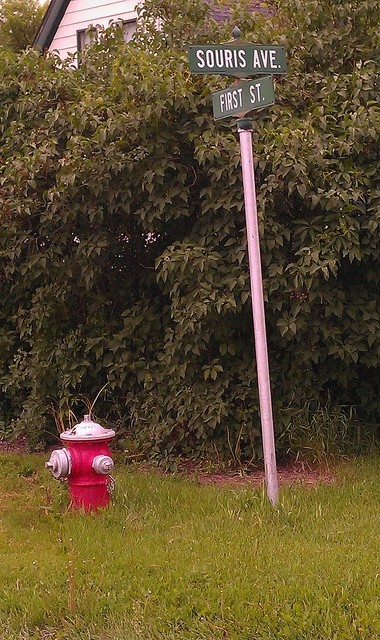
44;413;116;519
